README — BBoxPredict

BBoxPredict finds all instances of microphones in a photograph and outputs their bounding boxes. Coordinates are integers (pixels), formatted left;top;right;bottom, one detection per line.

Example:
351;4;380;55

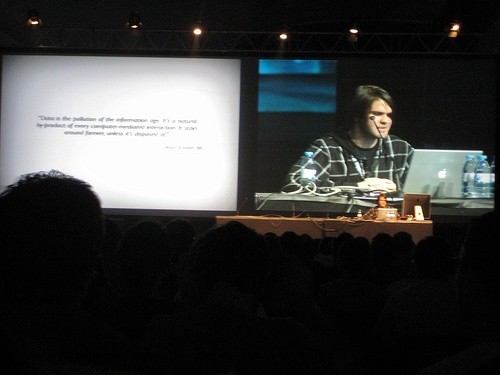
370;116;406;203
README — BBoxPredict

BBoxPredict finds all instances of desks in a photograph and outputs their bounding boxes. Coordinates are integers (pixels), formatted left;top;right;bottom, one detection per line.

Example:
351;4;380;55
215;215;434;245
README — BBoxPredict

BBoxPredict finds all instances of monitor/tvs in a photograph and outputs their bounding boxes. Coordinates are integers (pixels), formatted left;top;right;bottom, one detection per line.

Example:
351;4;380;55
402;193;431;221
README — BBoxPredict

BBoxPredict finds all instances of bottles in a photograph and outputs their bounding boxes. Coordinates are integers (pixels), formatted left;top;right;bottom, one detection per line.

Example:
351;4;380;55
461;153;496;200
356;209;362;220
385;211;395;222
301;152;316;186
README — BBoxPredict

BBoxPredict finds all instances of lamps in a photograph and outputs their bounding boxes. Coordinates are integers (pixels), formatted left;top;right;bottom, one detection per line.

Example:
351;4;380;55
126;12;144;30
24;9;43;30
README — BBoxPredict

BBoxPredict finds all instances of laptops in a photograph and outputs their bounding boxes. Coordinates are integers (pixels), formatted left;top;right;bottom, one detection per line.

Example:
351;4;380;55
377;208;397;221
400;149;484;198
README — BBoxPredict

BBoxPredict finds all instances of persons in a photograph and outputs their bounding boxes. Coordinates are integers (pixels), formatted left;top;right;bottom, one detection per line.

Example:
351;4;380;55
374;194;394;208
0;209;500;375
0;172;103;293
288;86;417;196
